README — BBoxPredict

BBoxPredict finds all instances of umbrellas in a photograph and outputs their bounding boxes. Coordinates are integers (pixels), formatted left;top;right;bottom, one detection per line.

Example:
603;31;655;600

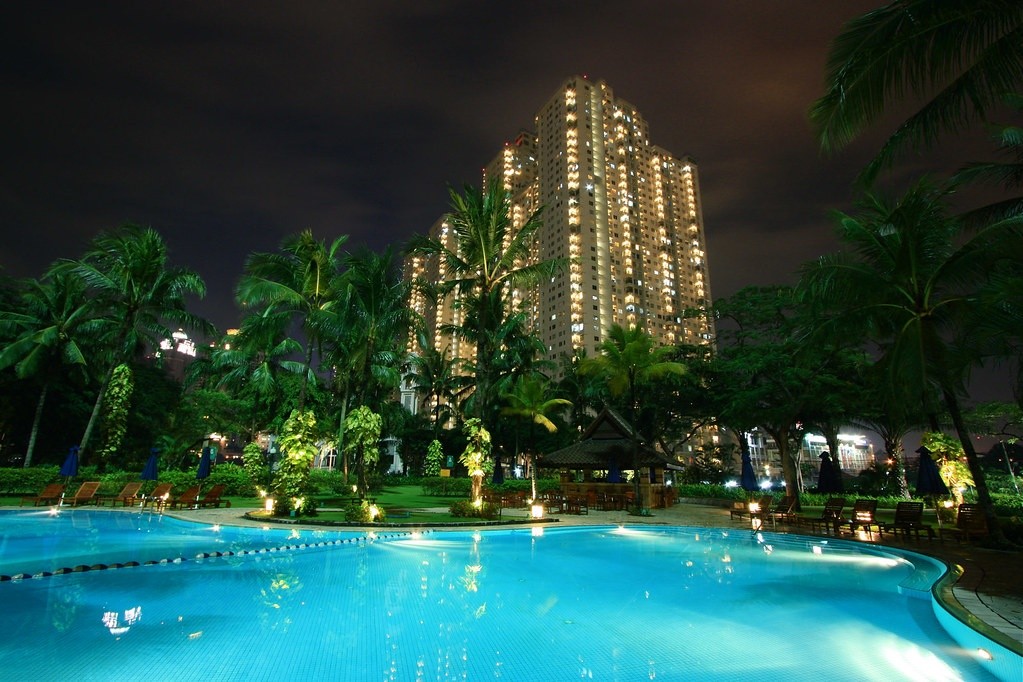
818;452;839;496
492;457;503;484
604;455;623;484
195;447;211;509
139;448;158;505
741;453;759;491
915;445;950;529
59;446;79;508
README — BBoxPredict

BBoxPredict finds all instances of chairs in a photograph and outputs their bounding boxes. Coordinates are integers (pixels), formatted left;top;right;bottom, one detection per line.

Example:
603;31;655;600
834;499;877;536
939;505;989;548
731;495;772;520
880;501;923;541
144;484;173;508
798;498;846;531
97;483;143;507
767;496;797;529
183;484;231;508
164;487;203;510
58;482;101;508
19;483;66;508
481;487;643;522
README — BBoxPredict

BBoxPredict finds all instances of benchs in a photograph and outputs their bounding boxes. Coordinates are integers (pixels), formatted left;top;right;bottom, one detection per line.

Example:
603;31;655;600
350;497;378;506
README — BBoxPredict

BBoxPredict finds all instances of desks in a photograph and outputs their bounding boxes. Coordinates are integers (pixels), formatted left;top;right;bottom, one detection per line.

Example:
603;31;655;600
905;524;932;543
609;494;627;510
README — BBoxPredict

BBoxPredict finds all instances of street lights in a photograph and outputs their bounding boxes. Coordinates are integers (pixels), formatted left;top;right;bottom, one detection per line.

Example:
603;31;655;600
204;414;223;437
